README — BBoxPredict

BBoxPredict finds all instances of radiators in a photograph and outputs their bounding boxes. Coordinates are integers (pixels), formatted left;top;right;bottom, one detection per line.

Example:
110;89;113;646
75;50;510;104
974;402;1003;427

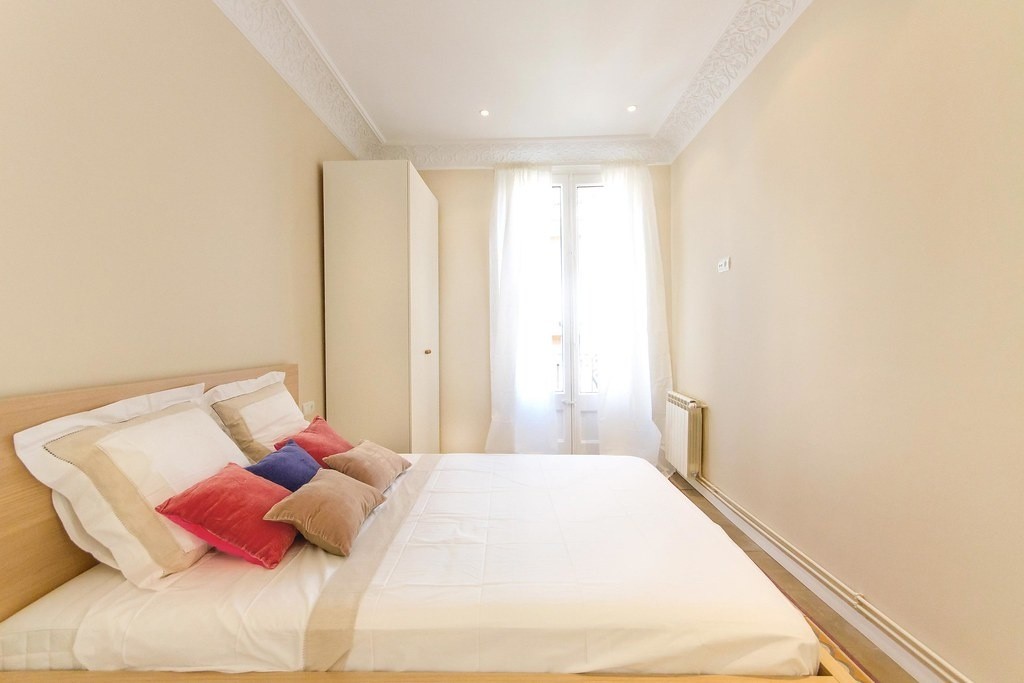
664;390;704;481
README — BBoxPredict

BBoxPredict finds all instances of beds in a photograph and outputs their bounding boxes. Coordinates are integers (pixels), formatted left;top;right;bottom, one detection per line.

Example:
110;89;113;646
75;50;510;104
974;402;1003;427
0;363;858;683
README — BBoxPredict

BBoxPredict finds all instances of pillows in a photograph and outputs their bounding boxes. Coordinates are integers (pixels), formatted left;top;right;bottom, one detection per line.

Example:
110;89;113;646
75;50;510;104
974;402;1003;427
273;413;354;470
154;463;299;569
262;468;386;559
323;439;413;493
244;440;323;491
201;371;311;466
12;382;252;589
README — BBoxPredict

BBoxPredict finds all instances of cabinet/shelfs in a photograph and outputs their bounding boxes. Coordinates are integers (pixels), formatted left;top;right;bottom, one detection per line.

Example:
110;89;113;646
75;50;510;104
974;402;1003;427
323;158;439;453
